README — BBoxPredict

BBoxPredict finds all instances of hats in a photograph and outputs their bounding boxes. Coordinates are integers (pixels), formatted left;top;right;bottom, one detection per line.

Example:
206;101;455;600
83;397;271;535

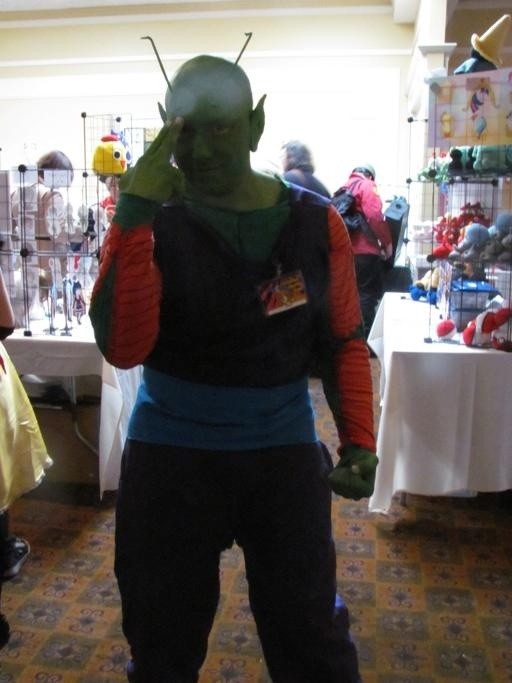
352;165;375;181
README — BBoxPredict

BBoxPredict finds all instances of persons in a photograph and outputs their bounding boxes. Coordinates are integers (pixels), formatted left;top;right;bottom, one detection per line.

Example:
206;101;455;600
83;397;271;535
0;269;53;579
279;140;394;359
90;56;378;683
99;176;120;221
10;150;85;290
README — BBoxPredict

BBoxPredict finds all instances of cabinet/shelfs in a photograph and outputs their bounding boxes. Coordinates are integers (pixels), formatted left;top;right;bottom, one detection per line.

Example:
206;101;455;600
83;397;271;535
401;81;512;348
1;110;144;335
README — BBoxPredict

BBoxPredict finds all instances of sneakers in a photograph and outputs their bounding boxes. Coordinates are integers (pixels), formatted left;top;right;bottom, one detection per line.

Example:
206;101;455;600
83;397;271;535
0;537;30;577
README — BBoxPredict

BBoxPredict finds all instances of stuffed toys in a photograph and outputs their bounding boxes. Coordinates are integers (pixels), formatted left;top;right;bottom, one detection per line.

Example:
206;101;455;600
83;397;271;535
453;14;511;74
408;145;512;350
94;134;127;176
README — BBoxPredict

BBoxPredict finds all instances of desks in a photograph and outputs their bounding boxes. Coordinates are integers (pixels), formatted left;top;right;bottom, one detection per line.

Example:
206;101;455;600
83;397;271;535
2;303;143;501
367;292;512;516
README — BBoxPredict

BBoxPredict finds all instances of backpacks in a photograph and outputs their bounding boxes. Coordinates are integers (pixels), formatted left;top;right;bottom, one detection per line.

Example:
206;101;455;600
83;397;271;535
332;191;363;233
